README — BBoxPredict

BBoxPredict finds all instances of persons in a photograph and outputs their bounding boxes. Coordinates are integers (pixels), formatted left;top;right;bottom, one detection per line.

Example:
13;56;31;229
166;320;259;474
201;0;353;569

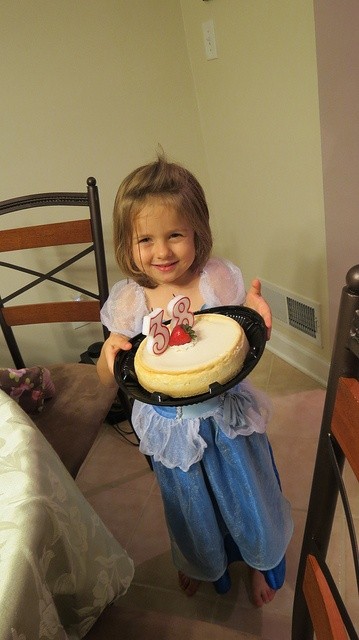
96;147;296;609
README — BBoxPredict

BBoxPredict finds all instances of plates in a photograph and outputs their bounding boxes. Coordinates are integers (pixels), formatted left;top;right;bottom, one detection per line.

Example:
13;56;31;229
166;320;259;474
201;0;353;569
113;305;266;407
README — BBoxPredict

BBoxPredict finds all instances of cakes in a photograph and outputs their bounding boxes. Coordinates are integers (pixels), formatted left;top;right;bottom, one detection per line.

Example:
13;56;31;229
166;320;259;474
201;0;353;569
135;312;251;398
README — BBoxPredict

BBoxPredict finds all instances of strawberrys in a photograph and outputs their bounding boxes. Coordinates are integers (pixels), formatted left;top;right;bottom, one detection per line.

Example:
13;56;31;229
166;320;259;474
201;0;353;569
170;324;198;344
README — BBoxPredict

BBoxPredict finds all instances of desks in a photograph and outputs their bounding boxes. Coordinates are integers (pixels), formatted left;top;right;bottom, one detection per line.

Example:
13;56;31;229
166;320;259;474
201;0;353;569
2;362;122;639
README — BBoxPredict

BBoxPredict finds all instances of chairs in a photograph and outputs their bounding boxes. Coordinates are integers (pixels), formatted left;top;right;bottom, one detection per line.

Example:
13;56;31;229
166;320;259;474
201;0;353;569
291;266;358;639
1;176;154;472
